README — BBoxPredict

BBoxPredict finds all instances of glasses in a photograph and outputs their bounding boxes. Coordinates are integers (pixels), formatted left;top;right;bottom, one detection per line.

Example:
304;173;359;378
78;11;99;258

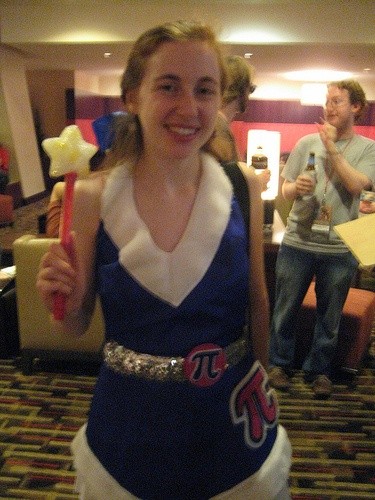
247;82;258;94
324;95;360;108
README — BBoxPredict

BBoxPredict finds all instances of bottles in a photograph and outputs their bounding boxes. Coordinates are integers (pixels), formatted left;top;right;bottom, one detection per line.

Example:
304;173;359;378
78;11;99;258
299;152;316;200
252;147;267;176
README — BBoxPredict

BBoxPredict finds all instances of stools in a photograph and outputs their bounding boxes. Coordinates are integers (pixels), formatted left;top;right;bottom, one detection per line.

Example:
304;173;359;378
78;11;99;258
298;282;375;389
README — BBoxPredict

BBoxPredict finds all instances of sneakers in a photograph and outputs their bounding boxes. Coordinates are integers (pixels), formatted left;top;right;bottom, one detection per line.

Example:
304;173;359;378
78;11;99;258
311;374;332;400
269;367;290;390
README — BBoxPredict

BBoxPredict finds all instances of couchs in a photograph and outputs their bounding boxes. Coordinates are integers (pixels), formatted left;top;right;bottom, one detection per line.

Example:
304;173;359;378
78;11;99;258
13;234;106;376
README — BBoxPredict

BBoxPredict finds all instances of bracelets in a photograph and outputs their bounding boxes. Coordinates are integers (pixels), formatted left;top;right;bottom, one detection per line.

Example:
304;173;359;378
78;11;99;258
324;147;342;159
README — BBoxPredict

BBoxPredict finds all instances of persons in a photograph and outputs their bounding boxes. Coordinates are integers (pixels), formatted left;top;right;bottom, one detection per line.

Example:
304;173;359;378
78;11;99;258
267;79;374;398
37;22;295;500
